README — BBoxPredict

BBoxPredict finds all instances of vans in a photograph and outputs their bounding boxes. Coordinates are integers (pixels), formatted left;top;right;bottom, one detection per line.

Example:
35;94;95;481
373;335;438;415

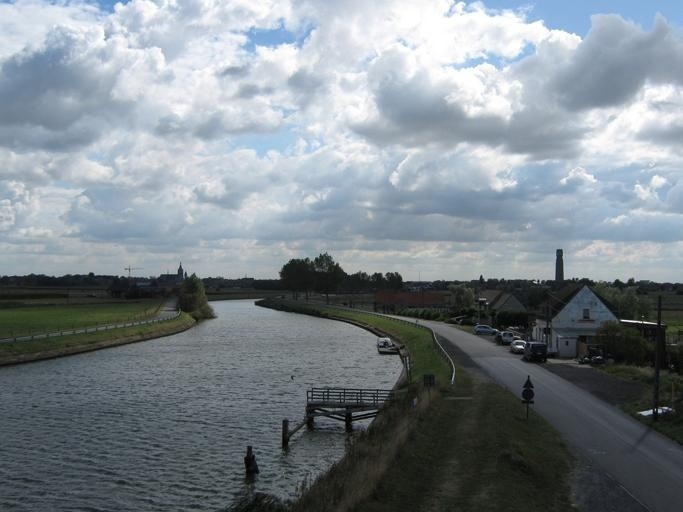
522;342;547;362
496;331;513;345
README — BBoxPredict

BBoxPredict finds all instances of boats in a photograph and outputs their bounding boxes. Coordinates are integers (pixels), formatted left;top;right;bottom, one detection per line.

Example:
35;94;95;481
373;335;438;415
377;336;392;347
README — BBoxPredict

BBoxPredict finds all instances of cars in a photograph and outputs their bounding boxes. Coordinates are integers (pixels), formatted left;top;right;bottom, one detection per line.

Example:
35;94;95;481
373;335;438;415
458;319;478;326
509;340;526;354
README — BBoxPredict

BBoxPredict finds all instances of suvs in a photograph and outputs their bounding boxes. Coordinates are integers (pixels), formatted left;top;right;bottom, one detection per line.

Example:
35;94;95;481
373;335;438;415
474;325;499;335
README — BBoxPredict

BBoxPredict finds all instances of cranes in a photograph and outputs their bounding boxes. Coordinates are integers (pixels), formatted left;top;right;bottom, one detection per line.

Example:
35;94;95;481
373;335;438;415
124;265;144;276
